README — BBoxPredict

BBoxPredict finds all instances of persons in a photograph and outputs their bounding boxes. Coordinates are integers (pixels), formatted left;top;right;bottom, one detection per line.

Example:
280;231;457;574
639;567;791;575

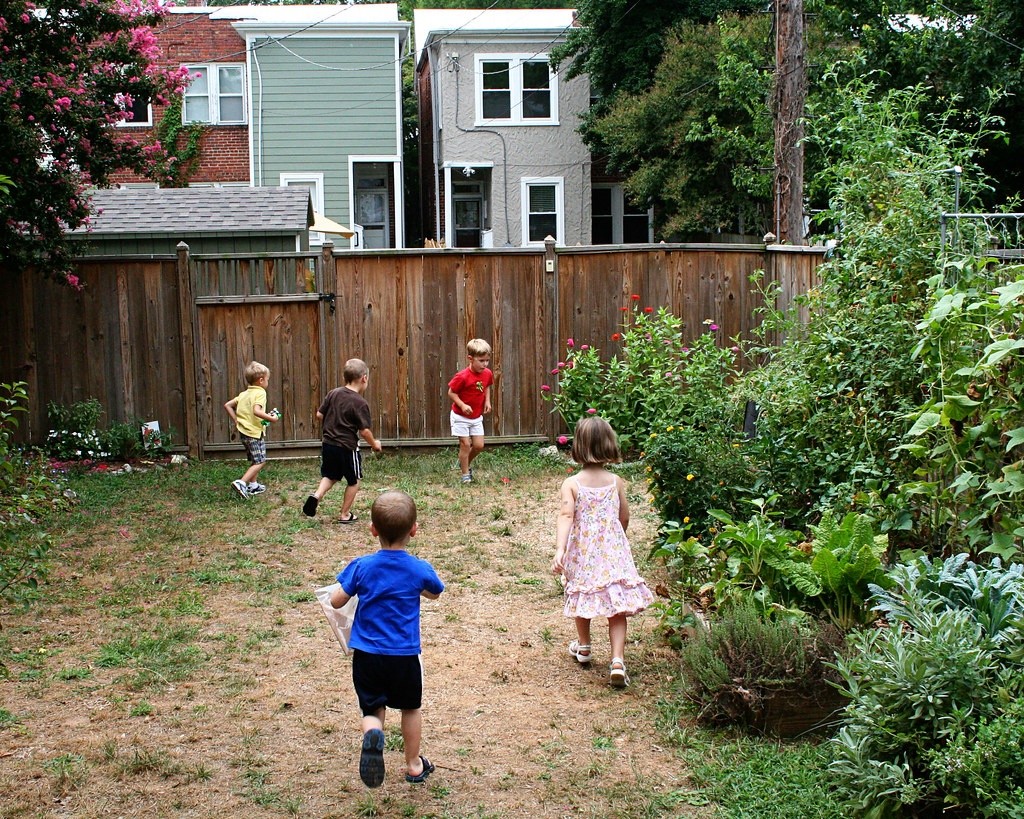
331;487;447;788
302;358;389;523
224;360;282;501
550;417;653;688
447;338;494;485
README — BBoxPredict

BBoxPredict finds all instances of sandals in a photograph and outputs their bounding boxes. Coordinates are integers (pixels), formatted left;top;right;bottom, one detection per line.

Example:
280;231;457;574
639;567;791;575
610;657;629;688
568;640;592;663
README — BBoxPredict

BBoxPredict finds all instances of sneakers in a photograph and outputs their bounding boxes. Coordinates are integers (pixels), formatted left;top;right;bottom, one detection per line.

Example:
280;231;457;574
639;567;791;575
461;473;471;484
246;483;266;495
231;479;250;500
467;467;475;482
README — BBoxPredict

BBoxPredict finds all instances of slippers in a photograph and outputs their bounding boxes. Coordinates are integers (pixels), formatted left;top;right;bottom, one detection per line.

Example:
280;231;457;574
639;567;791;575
338;512;358;522
360;728;386;788
303;495;319;517
406;755;435;782
